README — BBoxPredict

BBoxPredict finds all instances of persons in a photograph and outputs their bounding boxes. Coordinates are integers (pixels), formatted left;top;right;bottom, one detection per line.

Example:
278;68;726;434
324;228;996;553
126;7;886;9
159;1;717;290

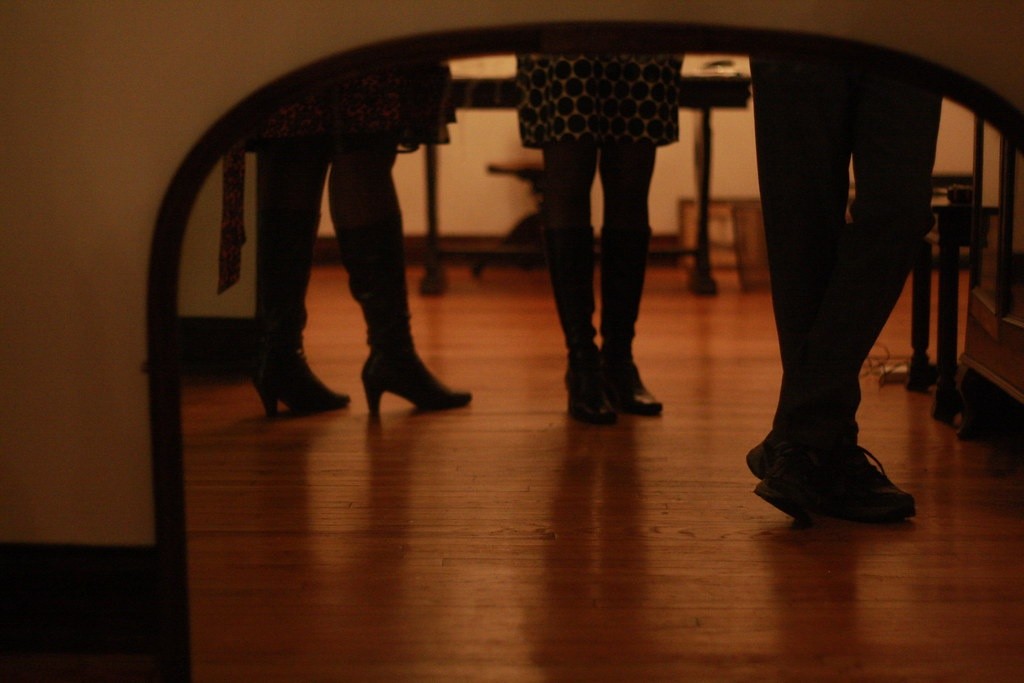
218;52;476;417
514;50;684;427
745;50;948;524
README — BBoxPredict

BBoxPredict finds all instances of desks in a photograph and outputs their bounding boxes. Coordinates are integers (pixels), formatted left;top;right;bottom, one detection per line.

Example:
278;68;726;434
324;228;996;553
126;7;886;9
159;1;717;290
419;73;754;296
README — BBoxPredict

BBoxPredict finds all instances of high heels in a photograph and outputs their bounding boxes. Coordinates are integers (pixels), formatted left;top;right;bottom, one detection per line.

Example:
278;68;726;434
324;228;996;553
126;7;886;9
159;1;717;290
362;346;472;415
247;341;350;416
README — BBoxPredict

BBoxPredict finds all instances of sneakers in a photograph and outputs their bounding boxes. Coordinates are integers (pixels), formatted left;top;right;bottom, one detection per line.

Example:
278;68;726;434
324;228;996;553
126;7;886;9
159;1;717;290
746;431;819;524
813;438;916;521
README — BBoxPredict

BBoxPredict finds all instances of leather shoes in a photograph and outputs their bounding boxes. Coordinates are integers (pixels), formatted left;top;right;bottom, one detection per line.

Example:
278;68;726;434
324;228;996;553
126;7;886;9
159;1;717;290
563;357;618;425
599;362;663;414
